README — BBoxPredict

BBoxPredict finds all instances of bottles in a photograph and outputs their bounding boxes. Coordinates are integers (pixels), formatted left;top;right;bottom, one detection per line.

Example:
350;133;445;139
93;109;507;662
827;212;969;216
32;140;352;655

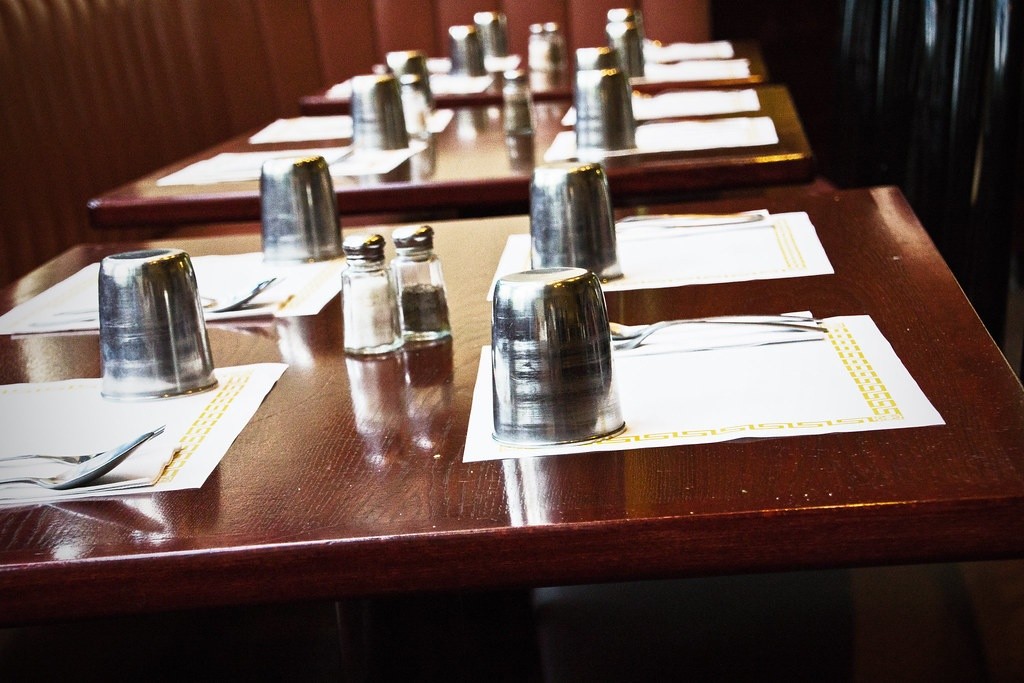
502;69;535;139
337;226;453;359
528;21;568;73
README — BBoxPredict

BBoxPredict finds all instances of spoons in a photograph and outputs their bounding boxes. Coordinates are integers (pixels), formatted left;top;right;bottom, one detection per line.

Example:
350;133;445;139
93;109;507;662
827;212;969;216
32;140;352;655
0;432;155;490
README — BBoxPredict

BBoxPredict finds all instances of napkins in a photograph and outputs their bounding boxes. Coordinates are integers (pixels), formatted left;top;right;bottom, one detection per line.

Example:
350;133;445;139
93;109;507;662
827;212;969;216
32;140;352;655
608;308;827;359
614;206;773;233
26;286;306;329
0;437;187;505
210;142;354;169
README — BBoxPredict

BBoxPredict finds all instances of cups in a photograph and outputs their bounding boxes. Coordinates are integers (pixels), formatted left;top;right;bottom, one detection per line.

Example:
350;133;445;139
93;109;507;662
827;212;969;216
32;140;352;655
575;46;620;69
98;248;218;398
472;11;508;56
491;267;636;445
351;73;410;150
529;160;623;281
260;155;348;262
574;69;638;150
629;354;636;357
449;24;488;75
604;22;649;77
387;51;438;112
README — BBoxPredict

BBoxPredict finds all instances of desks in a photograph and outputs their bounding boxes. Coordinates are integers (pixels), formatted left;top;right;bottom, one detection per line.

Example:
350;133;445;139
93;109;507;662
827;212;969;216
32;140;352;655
0;186;1024;683
297;44;770;115
81;84;817;232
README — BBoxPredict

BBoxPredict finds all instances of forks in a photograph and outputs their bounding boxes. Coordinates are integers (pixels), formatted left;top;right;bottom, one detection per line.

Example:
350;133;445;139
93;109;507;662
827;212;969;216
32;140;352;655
0;423;165;466
